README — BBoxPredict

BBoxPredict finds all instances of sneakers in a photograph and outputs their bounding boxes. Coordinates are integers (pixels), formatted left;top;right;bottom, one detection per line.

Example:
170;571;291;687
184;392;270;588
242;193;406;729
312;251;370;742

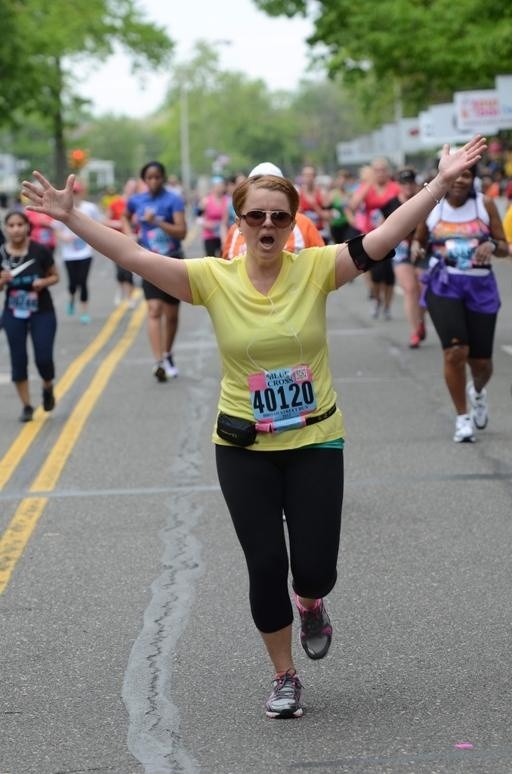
266;673;303;719
294;592;332;659
66;298;91;324
43;385;53;410
153;352;178;381
20;405;34;421
454;417;475;443
467;382;490;429
409;323;426;347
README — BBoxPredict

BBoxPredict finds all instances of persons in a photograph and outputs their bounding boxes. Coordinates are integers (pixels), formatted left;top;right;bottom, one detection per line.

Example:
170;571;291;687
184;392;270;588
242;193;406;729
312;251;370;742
407;146;508;443
292;138;512;349
20;133;488;720
1;160;246;423
221;162;325;260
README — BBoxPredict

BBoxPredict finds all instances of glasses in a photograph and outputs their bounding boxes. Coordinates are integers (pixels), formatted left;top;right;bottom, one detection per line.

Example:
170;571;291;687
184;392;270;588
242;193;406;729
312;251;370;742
241;210;295;229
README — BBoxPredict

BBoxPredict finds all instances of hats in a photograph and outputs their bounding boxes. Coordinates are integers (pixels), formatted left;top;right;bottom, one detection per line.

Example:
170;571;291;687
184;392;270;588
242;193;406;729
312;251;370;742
248;162;283;177
